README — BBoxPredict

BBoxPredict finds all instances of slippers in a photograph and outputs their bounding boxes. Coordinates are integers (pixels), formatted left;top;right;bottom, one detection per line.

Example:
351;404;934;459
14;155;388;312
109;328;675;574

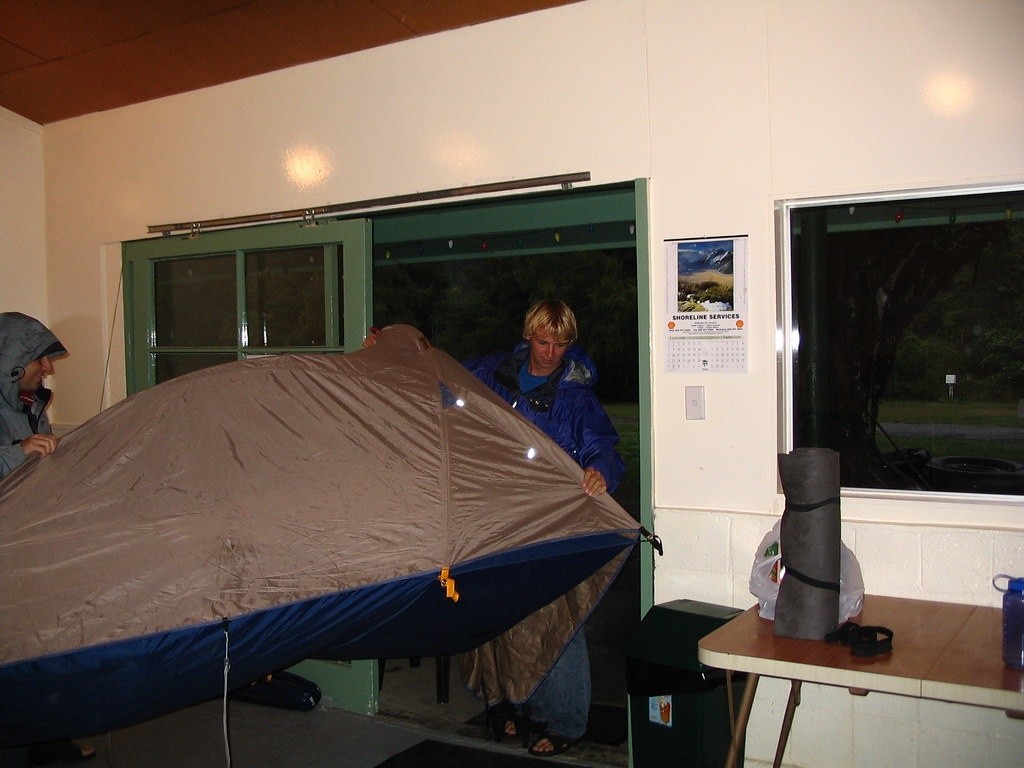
528;734;575;756
502;717;522;738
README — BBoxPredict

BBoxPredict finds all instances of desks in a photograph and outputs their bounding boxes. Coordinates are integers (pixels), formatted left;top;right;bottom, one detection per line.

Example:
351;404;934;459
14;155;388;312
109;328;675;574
699;594;1024;768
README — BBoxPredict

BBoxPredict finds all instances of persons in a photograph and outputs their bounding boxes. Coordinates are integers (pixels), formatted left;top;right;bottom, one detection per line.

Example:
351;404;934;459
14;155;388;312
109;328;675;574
0;311;99;768
361;300;625;756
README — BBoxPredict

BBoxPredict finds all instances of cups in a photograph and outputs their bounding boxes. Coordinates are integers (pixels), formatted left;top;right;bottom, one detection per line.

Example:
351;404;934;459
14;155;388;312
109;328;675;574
992;574;1024;668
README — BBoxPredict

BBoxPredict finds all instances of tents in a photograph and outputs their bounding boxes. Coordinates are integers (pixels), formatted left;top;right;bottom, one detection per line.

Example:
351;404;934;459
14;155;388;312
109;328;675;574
0;325;664;750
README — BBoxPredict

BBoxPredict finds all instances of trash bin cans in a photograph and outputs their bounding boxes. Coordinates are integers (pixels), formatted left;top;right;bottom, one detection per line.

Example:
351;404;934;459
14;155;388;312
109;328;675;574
628;600;750;768
927;455;1024;496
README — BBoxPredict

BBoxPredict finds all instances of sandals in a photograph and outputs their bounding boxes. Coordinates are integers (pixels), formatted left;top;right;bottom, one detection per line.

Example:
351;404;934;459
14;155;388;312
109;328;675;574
26;741;96;765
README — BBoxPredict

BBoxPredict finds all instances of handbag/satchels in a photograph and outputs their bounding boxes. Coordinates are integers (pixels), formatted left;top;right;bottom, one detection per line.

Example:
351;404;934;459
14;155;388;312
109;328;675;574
749;518;865;624
